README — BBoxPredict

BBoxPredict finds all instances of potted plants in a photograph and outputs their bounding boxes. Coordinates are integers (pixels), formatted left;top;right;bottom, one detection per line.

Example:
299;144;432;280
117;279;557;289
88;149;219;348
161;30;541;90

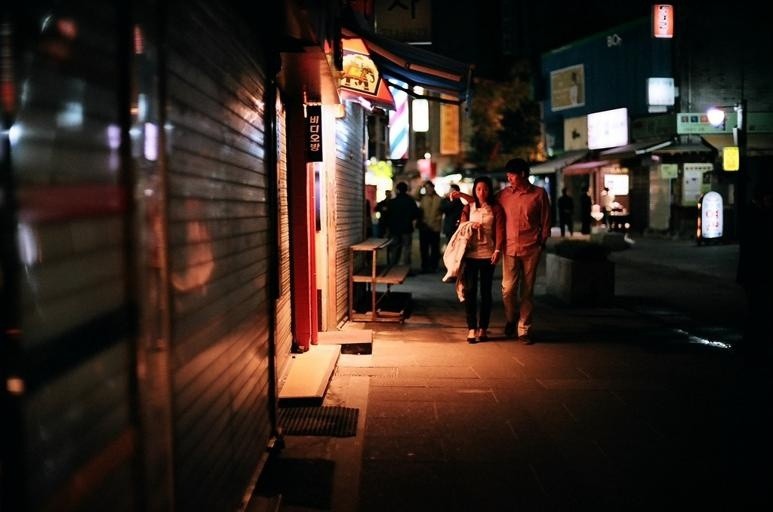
545;237;616;307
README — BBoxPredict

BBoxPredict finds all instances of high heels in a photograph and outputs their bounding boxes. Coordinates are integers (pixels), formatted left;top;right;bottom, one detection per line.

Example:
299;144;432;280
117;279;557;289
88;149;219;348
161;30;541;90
466;331;489;344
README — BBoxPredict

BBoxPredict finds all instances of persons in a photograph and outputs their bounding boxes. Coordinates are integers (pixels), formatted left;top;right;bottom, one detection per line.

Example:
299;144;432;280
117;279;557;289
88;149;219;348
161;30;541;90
449;157;552;345
580;185;592;234
604;187;615;229
373;190;392;239
558;187;575;237
438;184;464;244
460;176;506;344
413;180;442;273
384;182;423;265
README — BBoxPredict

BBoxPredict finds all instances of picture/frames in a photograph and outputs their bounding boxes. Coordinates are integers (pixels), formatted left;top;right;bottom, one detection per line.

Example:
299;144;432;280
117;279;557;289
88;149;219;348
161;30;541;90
550;64;586;113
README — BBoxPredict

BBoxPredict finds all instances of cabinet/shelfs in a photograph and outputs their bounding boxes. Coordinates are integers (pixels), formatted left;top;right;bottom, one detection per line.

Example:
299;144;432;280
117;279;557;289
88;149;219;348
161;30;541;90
348;236;411;324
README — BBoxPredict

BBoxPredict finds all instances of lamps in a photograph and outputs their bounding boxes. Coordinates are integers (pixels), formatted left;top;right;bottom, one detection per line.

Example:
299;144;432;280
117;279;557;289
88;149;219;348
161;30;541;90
303;91;345;119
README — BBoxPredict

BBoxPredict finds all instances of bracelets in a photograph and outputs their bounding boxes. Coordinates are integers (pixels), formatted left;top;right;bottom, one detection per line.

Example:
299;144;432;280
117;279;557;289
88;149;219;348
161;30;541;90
494;250;501;253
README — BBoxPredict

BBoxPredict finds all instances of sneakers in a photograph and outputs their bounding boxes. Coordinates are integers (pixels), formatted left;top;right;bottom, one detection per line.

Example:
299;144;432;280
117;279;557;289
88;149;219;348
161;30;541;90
504;317;529;343
